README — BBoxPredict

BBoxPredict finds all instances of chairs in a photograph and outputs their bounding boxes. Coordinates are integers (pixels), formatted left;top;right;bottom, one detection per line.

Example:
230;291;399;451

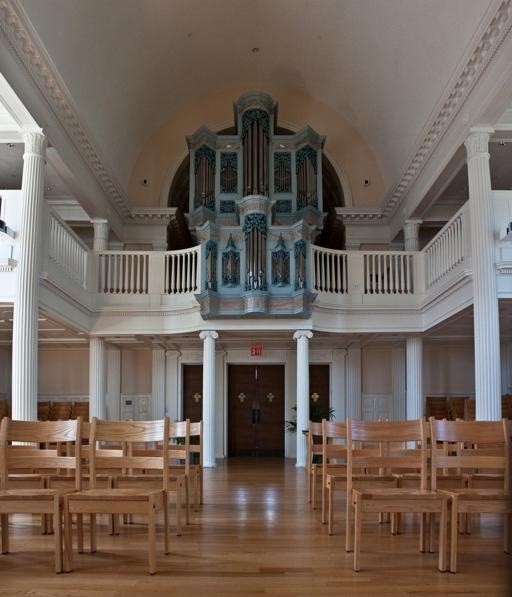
0;416;204;575
307;416;512;574
